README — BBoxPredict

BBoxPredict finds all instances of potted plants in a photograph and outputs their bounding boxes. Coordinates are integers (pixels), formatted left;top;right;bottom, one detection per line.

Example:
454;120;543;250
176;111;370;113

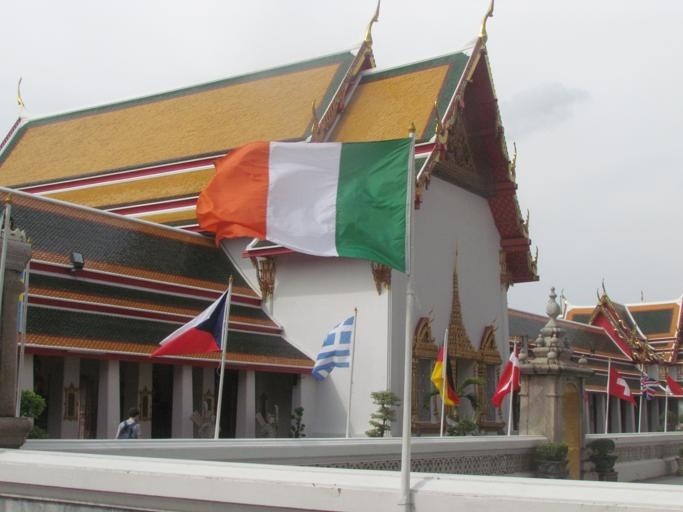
534;440;570;480
588;437;620;481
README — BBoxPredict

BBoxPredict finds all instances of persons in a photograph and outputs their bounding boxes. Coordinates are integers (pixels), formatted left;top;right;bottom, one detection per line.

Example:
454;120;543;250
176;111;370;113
113;406;143;438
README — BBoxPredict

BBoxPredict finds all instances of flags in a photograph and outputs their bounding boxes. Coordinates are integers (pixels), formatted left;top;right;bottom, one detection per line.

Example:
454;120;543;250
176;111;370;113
640;370;659;401
490;343;520;405
665;374;682;398
430;346;461;406
311;313;354;381
196;136;409;277
150;285;227;355
608;364;638;406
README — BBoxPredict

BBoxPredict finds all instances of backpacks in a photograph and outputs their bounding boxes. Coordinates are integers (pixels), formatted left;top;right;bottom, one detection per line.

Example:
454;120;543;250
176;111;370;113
117;420;138;439
